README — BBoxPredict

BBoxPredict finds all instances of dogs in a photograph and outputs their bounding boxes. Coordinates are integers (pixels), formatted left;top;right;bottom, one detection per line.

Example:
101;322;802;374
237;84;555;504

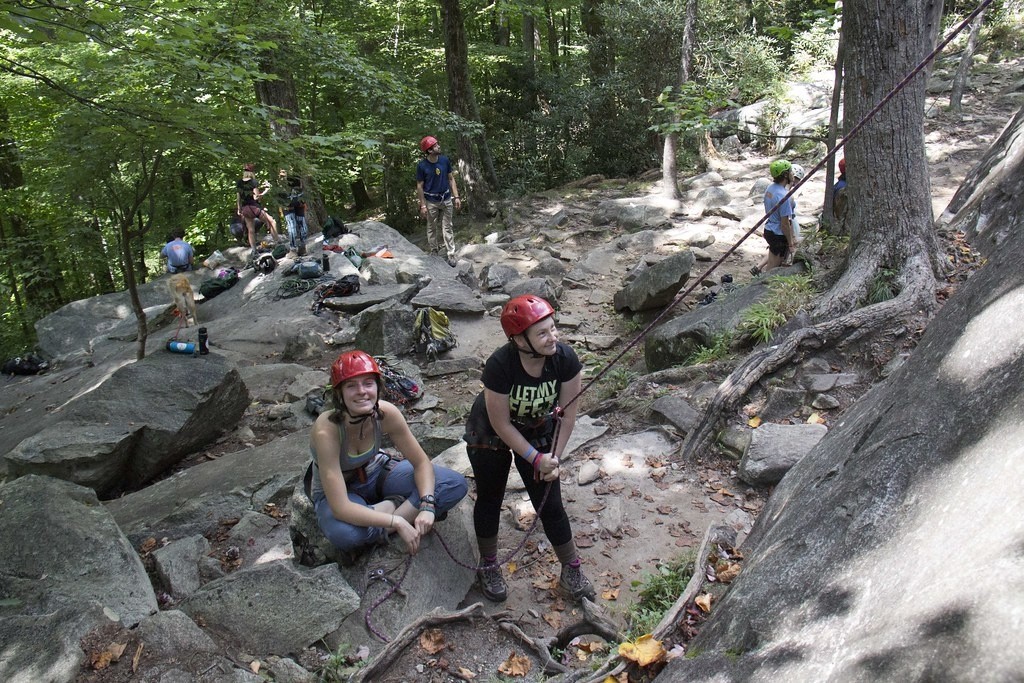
166;274;199;328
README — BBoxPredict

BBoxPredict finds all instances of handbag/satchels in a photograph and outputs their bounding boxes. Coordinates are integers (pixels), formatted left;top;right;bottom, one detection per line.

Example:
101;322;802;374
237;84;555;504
273;245;288;259
200;280;230;298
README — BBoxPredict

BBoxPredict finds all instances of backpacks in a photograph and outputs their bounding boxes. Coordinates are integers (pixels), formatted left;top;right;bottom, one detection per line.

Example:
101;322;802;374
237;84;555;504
411;307;456;360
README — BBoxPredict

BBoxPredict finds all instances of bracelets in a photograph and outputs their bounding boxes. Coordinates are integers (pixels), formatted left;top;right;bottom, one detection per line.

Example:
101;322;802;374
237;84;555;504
421;205;426;208
419;502;435;514
787;244;793;247
454;196;460;200
522;447;544;480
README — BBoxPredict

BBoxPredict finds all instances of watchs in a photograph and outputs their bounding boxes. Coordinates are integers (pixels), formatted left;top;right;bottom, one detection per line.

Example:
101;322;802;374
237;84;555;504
420;494;437;508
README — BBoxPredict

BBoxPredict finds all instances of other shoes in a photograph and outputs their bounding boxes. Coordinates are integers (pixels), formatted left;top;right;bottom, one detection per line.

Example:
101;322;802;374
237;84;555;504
748;266;761;276
447;254;457;267
430;248;439;256
352;528;390;567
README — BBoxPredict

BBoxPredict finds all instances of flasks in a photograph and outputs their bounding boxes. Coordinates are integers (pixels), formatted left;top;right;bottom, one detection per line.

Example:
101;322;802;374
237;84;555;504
322;254;330;271
166;339;196;353
198;327;210;355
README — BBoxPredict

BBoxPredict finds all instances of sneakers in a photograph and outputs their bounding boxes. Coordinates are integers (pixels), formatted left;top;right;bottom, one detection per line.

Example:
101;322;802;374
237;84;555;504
475;556;509;601
557;560;593;599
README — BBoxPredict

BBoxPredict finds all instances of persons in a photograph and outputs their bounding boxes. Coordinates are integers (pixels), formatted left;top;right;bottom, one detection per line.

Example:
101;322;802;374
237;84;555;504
160;227;194;274
416;136;461;267
216;222;228;242
230;207;264;247
750;159;805;279
462;295;597;603
277;161;308;256
237;164;281;257
833;157;847;196
309;350;469;556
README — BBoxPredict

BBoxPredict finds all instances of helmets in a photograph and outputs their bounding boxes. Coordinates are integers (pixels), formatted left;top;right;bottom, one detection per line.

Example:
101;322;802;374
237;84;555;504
330;349;381;389
420;135;437;151
243;163;254;171
770;160;791;178
501;294;553;340
791;163;804;179
838;158;846;173
